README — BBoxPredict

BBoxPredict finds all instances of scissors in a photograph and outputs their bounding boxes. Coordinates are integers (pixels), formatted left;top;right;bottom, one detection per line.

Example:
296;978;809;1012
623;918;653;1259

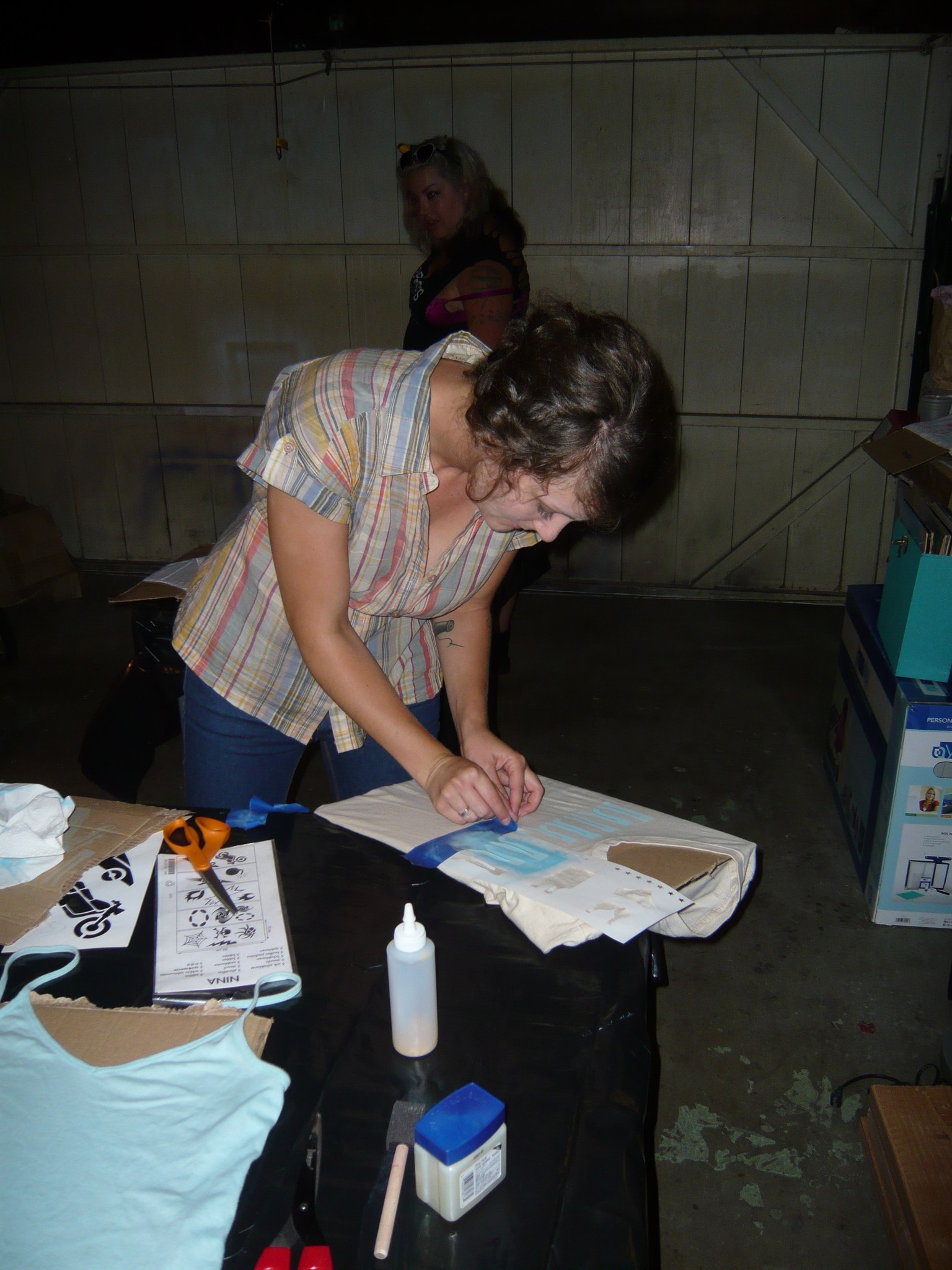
163;815;237;914
254;1113;332;1270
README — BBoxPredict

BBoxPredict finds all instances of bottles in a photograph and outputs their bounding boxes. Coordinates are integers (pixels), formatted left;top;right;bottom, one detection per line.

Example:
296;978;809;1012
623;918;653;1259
385;903;439;1059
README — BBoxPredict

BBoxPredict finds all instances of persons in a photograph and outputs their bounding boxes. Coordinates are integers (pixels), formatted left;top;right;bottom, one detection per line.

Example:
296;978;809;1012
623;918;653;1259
393;133;529;354
170;293;685;829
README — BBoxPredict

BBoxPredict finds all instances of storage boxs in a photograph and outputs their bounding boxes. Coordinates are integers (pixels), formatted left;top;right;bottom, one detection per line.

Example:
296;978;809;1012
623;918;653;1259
827;415;952;928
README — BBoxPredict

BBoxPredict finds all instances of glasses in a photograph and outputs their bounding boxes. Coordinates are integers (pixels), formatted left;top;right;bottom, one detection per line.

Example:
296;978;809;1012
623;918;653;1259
399;142;464;169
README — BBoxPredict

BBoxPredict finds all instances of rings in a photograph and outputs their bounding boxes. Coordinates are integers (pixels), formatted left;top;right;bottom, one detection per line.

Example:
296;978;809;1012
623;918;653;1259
460;805;470;817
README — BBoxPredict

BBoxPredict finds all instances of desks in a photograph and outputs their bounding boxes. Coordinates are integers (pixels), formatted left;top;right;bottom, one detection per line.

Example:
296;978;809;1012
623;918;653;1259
0;785;672;1270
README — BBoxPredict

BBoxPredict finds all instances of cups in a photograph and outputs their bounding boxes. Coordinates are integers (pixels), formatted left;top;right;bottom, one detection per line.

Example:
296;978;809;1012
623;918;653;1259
917;395;952;420
